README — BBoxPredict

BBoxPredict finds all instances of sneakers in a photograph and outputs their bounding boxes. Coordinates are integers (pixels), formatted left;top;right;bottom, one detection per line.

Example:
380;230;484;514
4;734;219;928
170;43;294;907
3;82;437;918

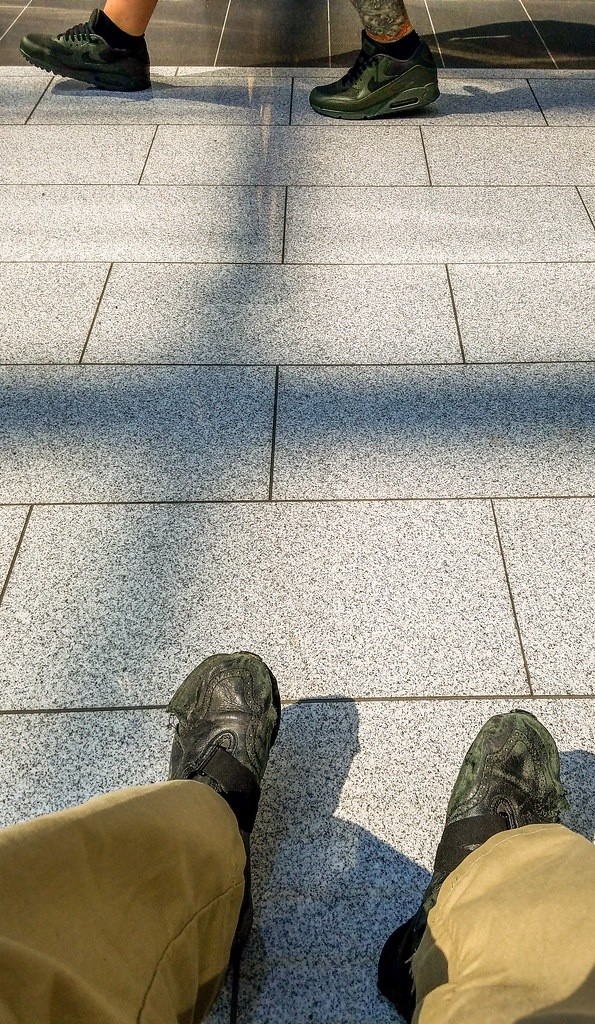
377;710;571;1024
165;652;280;976
20;8;151;92
309;32;440;120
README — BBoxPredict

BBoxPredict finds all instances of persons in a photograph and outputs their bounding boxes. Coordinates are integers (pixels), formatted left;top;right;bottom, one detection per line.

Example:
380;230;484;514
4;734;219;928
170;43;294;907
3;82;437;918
0;651;595;1024
20;0;441;121
